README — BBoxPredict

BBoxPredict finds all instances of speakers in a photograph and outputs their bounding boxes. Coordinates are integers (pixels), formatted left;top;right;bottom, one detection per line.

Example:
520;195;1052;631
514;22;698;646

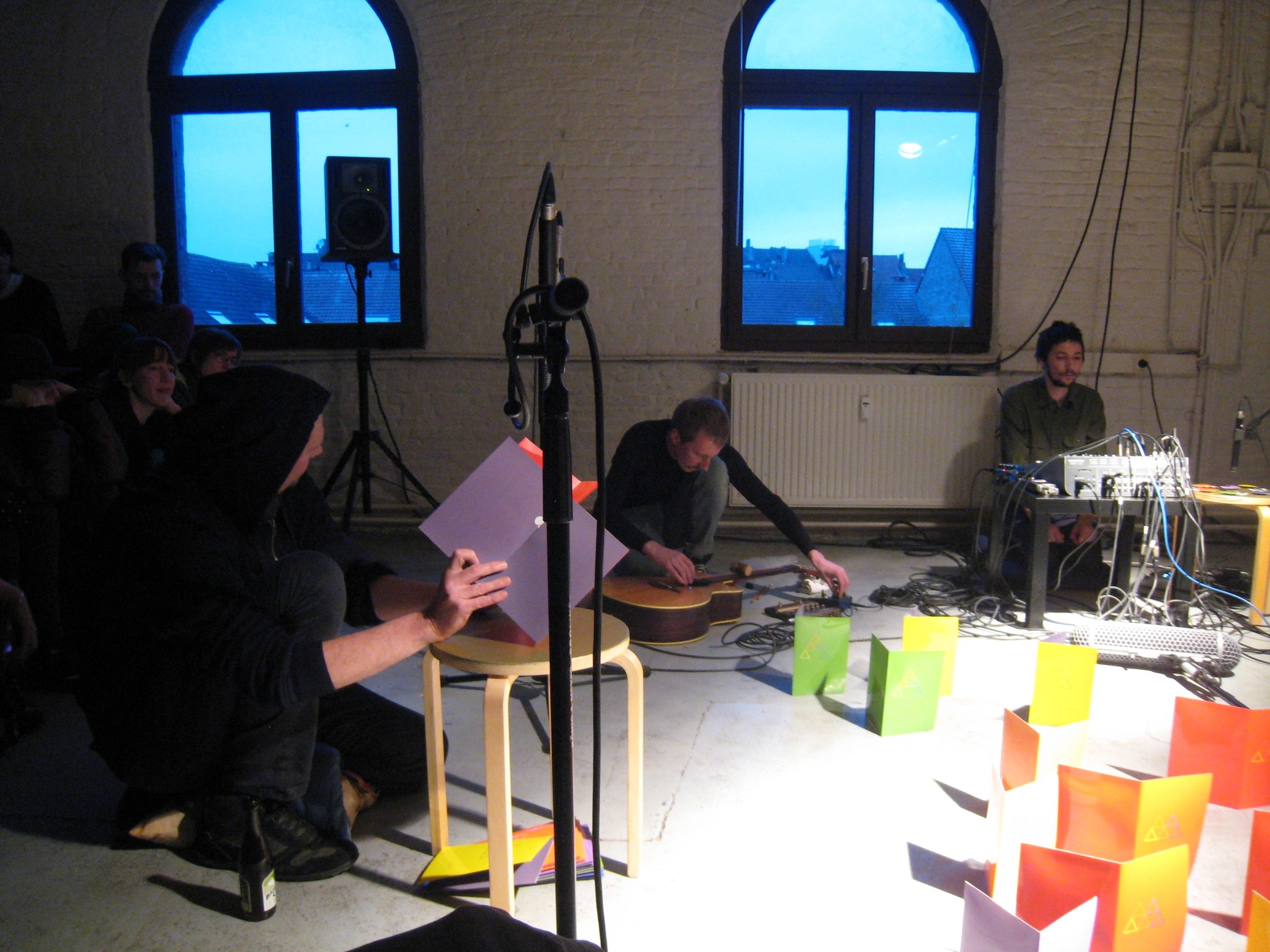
323;154;394;265
1069;622;1240;672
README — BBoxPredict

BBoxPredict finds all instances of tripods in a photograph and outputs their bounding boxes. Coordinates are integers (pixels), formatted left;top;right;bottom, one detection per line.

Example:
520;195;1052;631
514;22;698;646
311;267;441;534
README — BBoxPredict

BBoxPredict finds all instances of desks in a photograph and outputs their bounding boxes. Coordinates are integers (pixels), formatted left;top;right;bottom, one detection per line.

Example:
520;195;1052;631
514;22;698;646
985;475;1202;629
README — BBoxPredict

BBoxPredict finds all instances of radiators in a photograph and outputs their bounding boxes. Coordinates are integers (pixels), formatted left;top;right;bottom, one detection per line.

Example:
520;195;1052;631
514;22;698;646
729;372;998;507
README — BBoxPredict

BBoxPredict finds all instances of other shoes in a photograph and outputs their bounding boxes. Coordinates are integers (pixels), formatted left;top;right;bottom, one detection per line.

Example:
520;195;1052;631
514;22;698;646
193;793;360;883
696;565;710;574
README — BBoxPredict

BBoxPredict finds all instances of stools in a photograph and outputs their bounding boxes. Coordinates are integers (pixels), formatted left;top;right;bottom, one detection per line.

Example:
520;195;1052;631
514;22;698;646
1170;484;1270;625
422;604;644;877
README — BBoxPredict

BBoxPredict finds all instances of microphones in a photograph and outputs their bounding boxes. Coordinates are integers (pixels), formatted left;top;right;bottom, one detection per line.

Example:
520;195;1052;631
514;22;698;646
537;172;557;388
1139;359;1172;455
1229;400;1244;474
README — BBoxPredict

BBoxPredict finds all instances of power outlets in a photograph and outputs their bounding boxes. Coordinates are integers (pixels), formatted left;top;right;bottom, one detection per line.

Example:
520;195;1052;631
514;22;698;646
1134;355;1150;374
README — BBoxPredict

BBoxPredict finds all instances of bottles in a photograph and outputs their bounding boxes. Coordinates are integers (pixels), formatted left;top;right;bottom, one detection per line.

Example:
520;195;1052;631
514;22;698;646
239;796;277;922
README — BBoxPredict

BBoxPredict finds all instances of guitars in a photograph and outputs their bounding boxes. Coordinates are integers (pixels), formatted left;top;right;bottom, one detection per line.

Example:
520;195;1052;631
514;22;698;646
592;563;819;645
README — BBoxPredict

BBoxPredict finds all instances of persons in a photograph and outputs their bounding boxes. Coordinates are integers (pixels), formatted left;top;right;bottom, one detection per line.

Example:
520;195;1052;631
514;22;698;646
998;321;1106;583
0;242;512;880
593;397;851;597
